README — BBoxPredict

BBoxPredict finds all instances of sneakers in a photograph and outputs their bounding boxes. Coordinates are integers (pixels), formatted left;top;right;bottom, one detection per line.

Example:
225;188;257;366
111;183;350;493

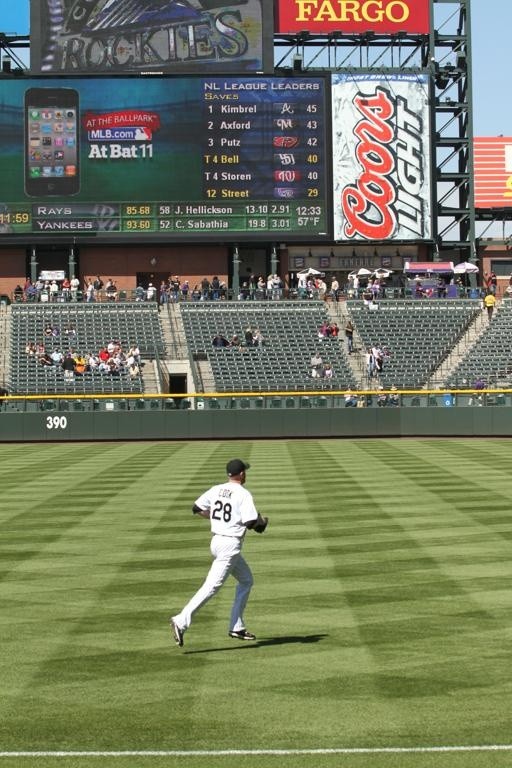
228;628;256;640
170;618;185;647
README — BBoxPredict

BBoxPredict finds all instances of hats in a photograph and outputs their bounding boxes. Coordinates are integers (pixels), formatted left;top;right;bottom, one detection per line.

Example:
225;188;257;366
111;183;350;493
226;458;251;478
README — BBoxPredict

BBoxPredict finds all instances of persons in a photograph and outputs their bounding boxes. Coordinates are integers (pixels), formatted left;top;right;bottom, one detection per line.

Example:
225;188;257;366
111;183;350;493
21;321;142;383
136;276;225;301
237;343;248;352
211;333;230;348
483;290;496;321
317;319;332;342
466;376;486;407
252;329;265;347
244;328;254;347
342;383;399;407
291;273;339;301
436;273;512;298
330;323;341;337
0;385;10;407
241;272;284;301
347;275;433;299
380;346;392;359
170;458;269;648
309;351;324;379
15;275;80;302
372;343;384;372
228;335;242;347
86;275;126;301
343;320;354;354
324;363;333;379
365;347;380;379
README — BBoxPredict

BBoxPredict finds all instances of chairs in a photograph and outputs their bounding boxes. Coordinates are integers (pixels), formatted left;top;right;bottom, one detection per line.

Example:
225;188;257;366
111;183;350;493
0;296;512;408
237;288;376;301
94;289;202;301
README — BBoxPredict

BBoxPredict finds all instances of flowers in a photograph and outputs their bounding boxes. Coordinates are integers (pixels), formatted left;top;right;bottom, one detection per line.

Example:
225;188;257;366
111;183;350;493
25;286;38;296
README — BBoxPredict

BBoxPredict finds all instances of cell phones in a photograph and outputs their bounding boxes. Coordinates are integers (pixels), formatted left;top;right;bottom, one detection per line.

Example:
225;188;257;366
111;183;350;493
24;88;80;197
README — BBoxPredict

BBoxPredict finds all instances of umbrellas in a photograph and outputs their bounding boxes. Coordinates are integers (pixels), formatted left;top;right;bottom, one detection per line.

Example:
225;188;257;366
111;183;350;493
452;262;478;287
368;265;396;281
347;266;374;286
296;267;326;282
